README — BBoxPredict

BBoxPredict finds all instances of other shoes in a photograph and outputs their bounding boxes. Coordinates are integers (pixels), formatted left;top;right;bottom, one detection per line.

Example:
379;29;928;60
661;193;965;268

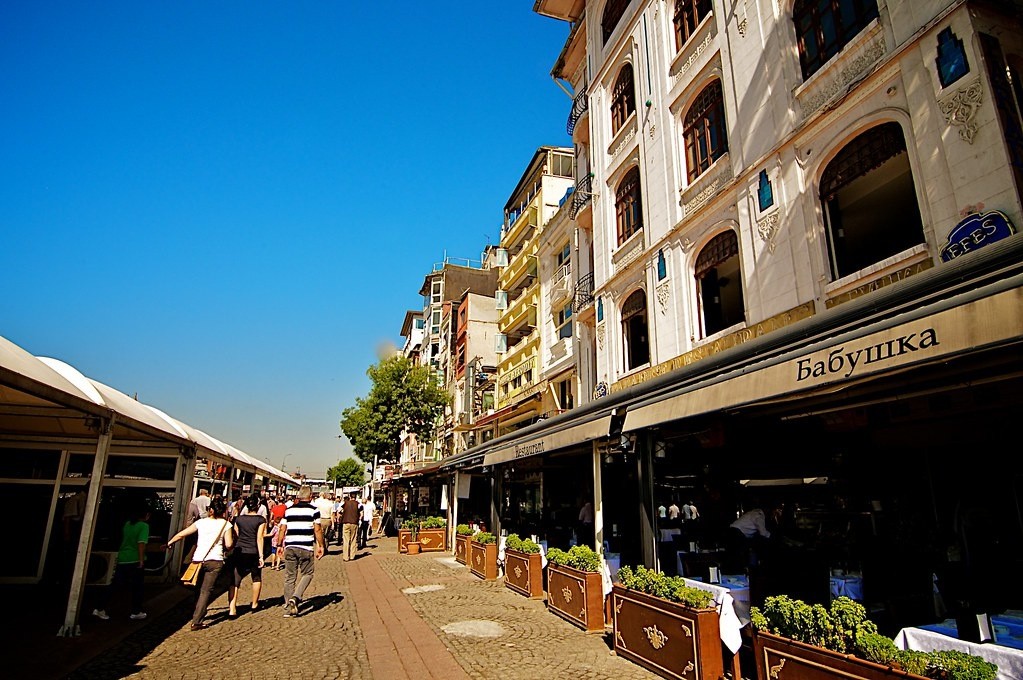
271;566;275;570
276;567;280;571
289;597;299;614
229;613;237;619
251;603;260;612
191;623;209;631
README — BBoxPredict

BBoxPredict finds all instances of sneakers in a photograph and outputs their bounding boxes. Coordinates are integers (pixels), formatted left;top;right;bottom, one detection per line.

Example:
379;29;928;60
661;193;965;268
93;609;111;619
131;612;146;620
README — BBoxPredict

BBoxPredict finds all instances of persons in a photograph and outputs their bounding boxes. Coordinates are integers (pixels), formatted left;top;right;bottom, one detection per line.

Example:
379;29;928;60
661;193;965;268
659;500;699;519
63;480;91;544
577;497;594;546
165;489;376;630
93;504;154;620
721;503;773;566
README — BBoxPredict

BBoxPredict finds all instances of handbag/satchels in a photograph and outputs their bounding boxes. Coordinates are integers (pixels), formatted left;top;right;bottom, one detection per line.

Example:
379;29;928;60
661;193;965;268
369;527;372;536
180;562;201;586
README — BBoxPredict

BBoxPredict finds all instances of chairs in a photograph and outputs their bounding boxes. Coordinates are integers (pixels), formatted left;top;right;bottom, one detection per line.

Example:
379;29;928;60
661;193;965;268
683;553;723;582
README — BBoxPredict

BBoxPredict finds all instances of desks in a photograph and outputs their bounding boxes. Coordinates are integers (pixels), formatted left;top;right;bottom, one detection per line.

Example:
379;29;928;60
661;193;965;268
535;540;578;569
892;610;1023;680
689;574;751;680
598;553;622;603
831;576;866;601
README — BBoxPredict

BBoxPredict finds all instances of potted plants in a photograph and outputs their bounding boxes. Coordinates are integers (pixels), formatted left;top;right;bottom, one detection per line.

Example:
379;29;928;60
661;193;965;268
454;524;476;567
418;516;447;552
398;514;419;553
470;531;498;582
502;533;546;601
610;565;724;680
749;595;998;680
546;544;605;635
407;522;421;555
